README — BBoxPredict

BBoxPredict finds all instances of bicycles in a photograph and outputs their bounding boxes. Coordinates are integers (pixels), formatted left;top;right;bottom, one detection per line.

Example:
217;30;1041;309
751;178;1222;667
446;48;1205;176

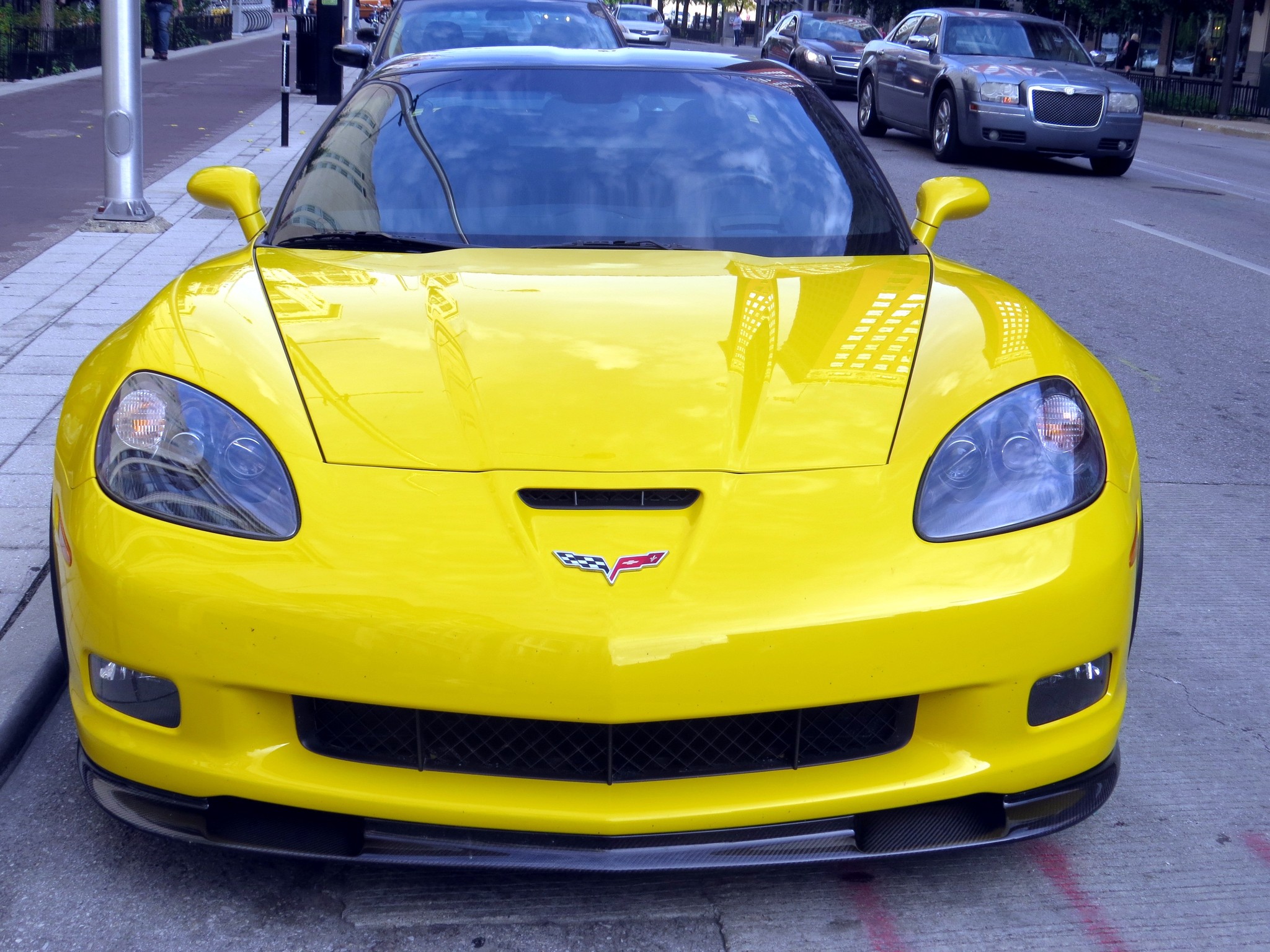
362;0;389;44
212;0;272;34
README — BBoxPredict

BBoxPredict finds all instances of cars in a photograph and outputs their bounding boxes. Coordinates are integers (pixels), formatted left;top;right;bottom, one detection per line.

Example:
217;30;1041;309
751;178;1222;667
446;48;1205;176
332;0;628;93
610;3;671;50
760;10;883;101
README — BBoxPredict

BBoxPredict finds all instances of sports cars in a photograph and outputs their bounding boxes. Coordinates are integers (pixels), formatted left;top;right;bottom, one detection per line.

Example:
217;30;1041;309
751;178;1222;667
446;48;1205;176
44;41;1150;876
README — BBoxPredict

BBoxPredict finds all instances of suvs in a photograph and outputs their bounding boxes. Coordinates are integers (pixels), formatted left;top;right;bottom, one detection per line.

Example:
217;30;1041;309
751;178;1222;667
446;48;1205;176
306;0;393;24
855;6;1145;178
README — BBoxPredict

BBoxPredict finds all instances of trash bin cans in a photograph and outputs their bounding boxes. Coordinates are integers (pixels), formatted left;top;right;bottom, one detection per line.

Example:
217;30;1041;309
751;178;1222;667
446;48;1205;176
291;14;317;96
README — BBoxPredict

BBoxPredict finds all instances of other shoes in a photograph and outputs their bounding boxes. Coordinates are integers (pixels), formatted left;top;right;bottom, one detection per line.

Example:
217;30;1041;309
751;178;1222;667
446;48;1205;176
152;52;167;61
734;45;739;47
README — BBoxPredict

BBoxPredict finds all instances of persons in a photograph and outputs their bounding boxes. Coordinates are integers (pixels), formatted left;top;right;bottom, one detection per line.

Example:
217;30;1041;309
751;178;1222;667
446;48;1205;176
143;0;183;60
1121;33;1141;80
271;0;305;16
732;11;742;48
878;27;886;39
693;12;700;30
